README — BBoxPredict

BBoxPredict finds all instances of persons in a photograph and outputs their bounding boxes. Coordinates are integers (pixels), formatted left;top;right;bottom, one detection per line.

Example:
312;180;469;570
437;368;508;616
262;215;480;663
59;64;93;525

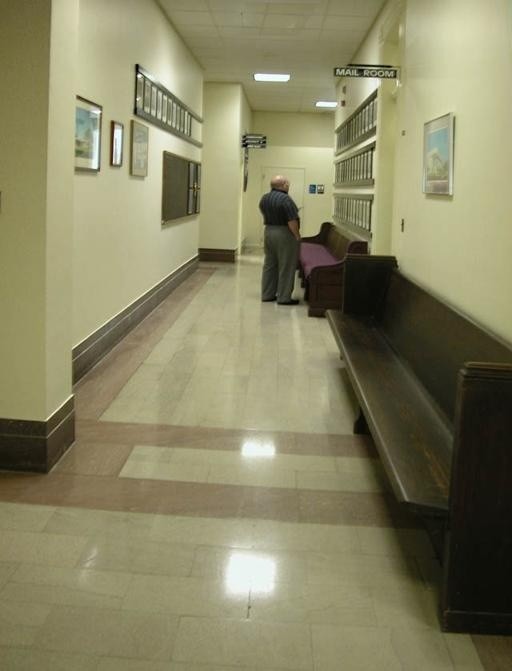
259;174;300;306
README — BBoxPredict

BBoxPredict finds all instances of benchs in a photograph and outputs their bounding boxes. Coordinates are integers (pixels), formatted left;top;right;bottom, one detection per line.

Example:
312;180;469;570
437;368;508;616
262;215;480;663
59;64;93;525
295;223;511;635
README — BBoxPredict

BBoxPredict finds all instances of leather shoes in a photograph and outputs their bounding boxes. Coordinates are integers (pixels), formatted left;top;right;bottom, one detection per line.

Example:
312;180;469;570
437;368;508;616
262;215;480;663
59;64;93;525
262;296;299;305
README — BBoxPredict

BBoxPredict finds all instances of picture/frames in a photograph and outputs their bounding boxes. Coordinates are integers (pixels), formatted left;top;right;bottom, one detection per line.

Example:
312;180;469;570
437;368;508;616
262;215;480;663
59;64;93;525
420;112;453;195
75;95;151;177
133;66;191;139
188;160;202;215
333;88;377;242
